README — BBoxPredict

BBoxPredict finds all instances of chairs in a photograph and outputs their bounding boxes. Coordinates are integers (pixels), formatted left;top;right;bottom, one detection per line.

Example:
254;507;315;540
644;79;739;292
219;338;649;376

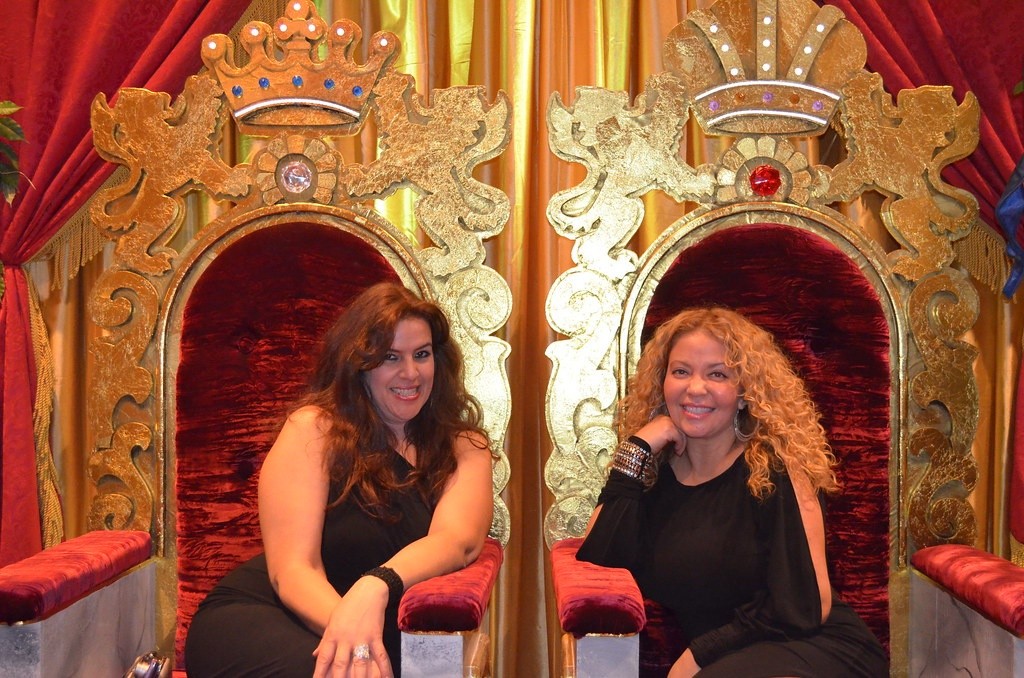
541;200;1024;677
0;202;507;678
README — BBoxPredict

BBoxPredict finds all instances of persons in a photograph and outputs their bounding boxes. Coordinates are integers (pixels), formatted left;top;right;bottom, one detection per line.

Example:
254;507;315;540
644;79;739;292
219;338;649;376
576;307;890;678
185;285;500;678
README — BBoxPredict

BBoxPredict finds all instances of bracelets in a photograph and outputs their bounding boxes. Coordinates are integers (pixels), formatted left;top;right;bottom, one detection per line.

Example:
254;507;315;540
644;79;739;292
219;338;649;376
359;565;405;603
611;440;659;488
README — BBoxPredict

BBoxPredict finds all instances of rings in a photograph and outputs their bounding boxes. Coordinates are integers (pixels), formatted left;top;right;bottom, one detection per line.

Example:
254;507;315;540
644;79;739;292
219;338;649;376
353;643;370;659
